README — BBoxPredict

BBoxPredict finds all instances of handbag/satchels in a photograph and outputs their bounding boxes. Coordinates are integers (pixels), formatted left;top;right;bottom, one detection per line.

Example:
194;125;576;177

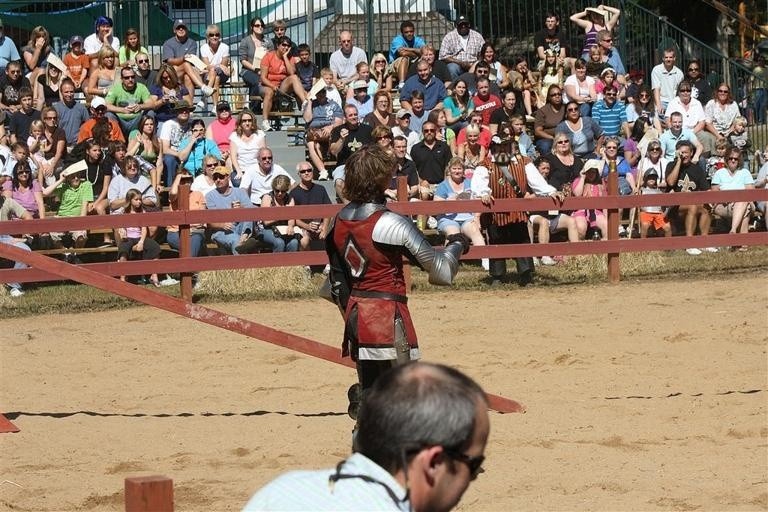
273;222;303;241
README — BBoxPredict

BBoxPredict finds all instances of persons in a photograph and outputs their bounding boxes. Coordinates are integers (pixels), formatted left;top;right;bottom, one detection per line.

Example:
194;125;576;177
347;80;374;124
422;111;457;152
591;85;631;140
652;46;686;131
257;37;308;131
368;125;393;148
626;85;662;138
308;68;344;109
294;43;318;92
329;104;374;205
564;58;599;118
470;43;511;88
301;87;345;181
88;45;121;96
242;359;489;512
177;119;223;180
83;18;120;56
23;26;55;90
637;140;670;192
106;155;160;245
712;147;756;252
415;45;452;88
0;19;20;91
330;31;368;92
661;112;703;165
167;168;208;275
570;159;609;242
27;121;53;167
537;131;586;194
6;142;40;183
705;84;743;151
396;62;448;120
749;55;768;123
554;101;607;161
507;114;538;163
39;106;69;177
725;117;756;164
705;138;733;178
459;59;502;102
254;175;302;254
65;35;90;88
402;89;431;133
228;109;266;187
444;80;474;130
117;29;150;69
240;147;297;207
239;17;274;113
86;121;115;160
347;62;380;103
457;124;487;169
595;29;626;84
441;13;485;79
457;108;492;152
0;175;34;294
633;168;670;240
490;92;522;135
328;145;472;418
163;19;217;107
677;60;712;109
149;63;194;138
362;92;396;135
539;49;564;110
623;117;654;182
584;46;613;76
511;59;536;122
410;121;452;192
42;161;93;264
595;137;640;236
8;87;40;148
592;67;621;97
666;140;718;255
289;162;332;274
158;101;196;185
270;20;298;54
2;158;47;251
430;157;487;268
528;157;582;266
474;134;566;286
472;78;502;124
54;76;91;151
664;80;710;161
366;53;393;113
205;100;237;170
78;96;125;156
535;11;579;77
105;67;157;128
189;154;232;196
204;166;258;257
389;21;426;94
0;60;31;112
533;85;568;157
389;109;419;155
372;126;418;203
753;147;768;232
38;60;77;111
570;5;621;63
112;190;161;288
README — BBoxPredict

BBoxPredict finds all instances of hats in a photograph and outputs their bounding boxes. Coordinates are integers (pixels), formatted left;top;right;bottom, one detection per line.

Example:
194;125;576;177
491;133;513;145
174;19;187;29
644;168;658;179
353;79;370;89
171;100;196;113
91;97;106;108
395;109;412;119
457;14;470;25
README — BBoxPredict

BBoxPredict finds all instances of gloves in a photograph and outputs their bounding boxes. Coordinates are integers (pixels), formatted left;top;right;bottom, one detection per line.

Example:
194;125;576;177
446;232;470;254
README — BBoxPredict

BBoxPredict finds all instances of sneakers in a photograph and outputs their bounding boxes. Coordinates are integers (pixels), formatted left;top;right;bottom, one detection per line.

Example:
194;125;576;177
700;247;718;253
262;120;273;132
686;248;701;255
519;277;533;288
10;288;25;297
318;170;329;181
323;264;330;275
235;238;255;255
541;257;557;266
619;224;626;236
482;258;490;272
202;84;217;97
398;83;405;93
197;101;208;110
533;258;539;267
491;277;505;288
211;106;217;116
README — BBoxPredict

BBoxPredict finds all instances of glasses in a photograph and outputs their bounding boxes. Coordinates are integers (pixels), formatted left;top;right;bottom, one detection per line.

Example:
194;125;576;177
219;108;229;112
423;129;436;133
16;171;30;175
558;140;569;144
396;441;485;484
9;70;20;74
275;190;286;194
638;93;650;100
603;39;612;43
261;156;273;161
213;175;225;181
44;117;56;120
299;168;313;174
139;59;149;64
606;147;617;149
123;75;136;80
242;119;253;123
279;42;291;47
192;128;205;132
605;93;616;96
126;164;138;170
567;108;579;113
207;163;218;167
477;70;489;74
254;24;265;28
650;149;659;151
717;90;729;93
679;89;691;92
209;33;220;37
727;157;739;161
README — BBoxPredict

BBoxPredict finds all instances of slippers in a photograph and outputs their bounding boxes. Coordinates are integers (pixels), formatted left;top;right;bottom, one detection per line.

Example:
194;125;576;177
149;279;162;288
